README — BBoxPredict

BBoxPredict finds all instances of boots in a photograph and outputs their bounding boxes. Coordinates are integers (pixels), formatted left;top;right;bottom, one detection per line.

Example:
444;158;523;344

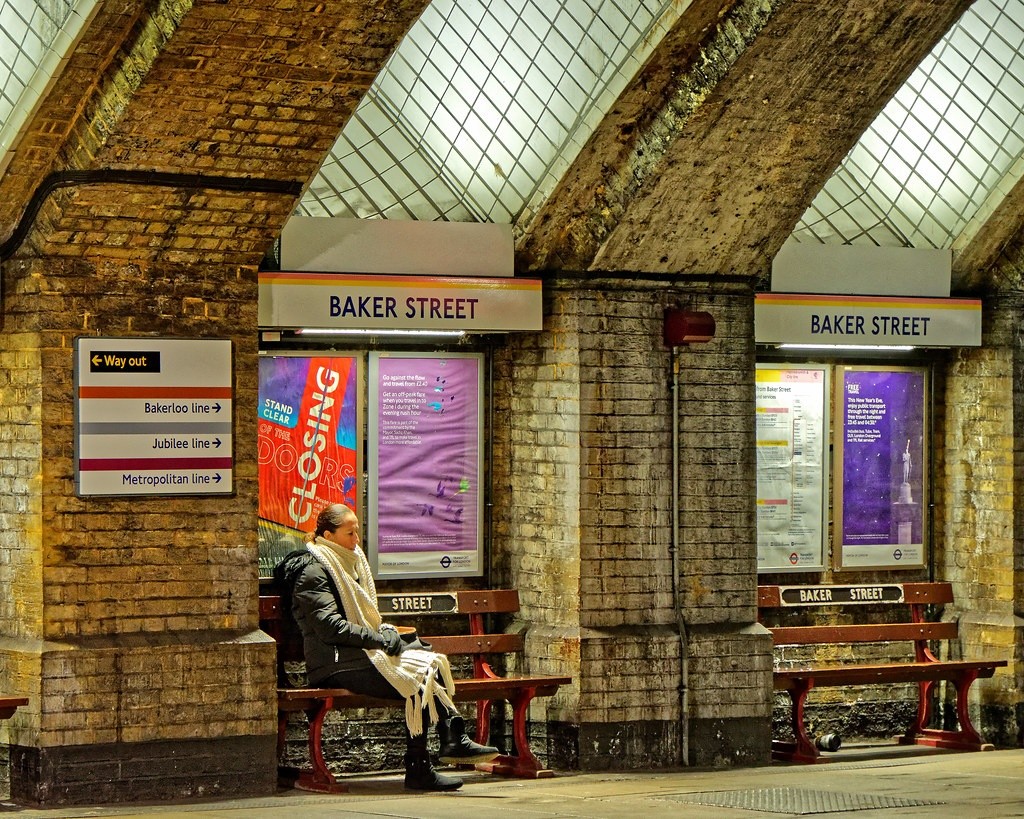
404;753;463;791
438;716;498;765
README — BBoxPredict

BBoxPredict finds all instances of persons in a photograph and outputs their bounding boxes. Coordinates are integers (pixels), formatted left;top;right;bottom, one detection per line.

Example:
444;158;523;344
283;503;499;792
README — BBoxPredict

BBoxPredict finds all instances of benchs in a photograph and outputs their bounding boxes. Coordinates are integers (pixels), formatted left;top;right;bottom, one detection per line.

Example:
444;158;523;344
259;590;572;794
757;582;1008;763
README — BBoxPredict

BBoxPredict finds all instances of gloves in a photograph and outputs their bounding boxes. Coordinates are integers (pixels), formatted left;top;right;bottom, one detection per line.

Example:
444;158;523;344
379;623;402;656
400;639;408;647
